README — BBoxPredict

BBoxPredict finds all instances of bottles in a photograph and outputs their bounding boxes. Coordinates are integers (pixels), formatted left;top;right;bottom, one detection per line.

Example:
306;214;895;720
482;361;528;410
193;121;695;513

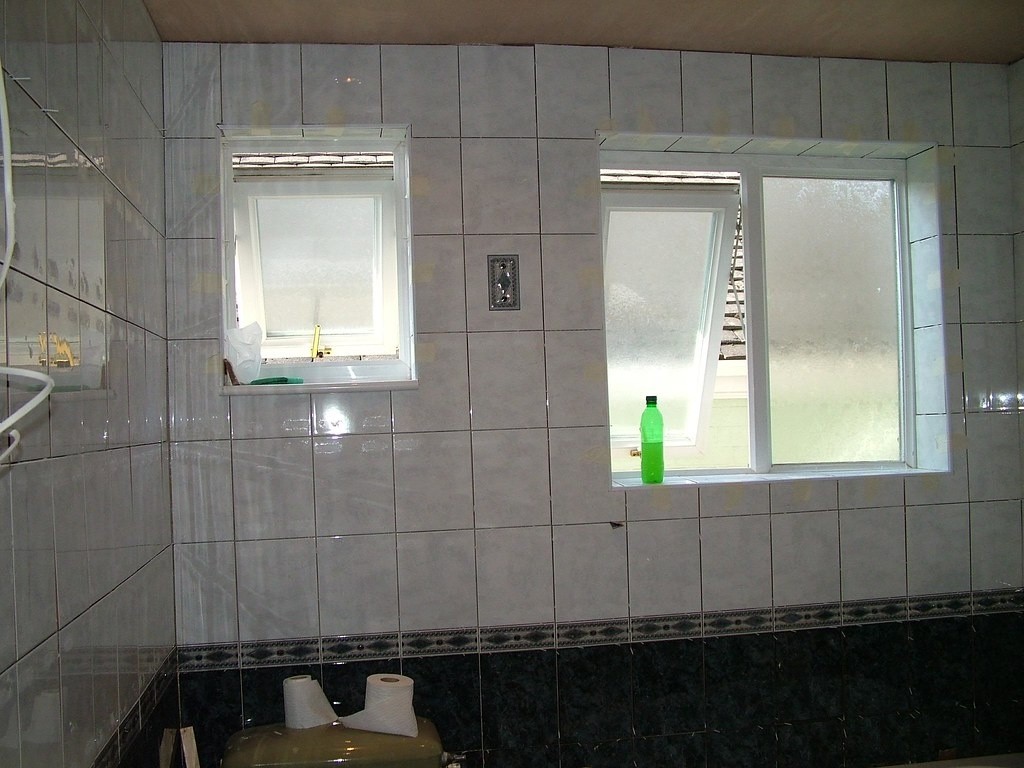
639;395;666;485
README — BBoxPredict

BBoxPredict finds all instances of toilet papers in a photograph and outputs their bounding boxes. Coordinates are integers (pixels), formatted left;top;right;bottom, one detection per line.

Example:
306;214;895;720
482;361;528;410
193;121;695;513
282;673;420;738
